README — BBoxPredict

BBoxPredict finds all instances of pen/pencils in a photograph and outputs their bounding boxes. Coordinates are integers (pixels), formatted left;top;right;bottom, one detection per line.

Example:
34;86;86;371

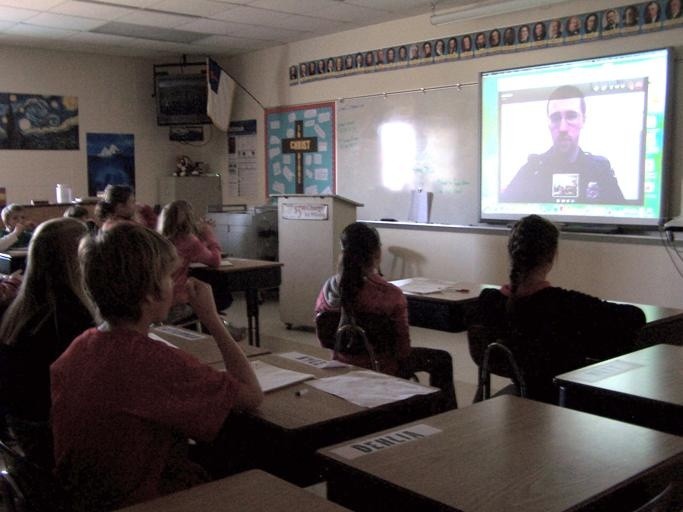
248;352;272;357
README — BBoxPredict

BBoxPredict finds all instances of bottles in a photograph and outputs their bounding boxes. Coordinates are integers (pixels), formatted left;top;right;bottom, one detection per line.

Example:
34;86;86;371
56;182;73;204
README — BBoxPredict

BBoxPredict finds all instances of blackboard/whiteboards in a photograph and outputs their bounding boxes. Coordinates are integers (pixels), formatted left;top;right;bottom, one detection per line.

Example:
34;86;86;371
335;82;482;229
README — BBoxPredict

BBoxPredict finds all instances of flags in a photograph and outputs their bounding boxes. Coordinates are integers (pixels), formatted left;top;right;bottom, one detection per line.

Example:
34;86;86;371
206;54;236;134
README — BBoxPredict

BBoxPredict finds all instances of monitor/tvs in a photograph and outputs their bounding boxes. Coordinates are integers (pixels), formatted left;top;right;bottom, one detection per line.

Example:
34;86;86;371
155;74;209;121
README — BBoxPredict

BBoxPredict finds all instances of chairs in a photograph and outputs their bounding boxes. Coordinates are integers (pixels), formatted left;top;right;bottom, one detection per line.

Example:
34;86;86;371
0;440;45;510
316;306;400;370
467;323;528;399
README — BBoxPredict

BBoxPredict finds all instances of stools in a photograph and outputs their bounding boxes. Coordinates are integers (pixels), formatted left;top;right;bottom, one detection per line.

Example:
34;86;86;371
390;245;426;278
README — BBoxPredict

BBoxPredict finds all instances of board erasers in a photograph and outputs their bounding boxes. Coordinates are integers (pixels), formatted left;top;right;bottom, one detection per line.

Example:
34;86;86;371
381;219;396;221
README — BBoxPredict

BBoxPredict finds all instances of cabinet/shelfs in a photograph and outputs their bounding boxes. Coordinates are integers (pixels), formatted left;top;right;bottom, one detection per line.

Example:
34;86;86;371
207;211;277;258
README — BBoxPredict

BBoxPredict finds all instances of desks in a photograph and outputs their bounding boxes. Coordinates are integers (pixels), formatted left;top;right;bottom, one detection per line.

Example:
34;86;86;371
213;350;441;472
551;345;683;438
313;393;682;512
188;254;285;343
605;298;682;345
151;321;271;364
122;469;350;512
389;277;502;401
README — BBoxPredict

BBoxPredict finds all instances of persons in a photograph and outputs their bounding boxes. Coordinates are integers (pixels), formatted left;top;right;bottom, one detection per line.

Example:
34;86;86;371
314;222;457;418
477;215;646;406
1;184;265;512
499;86;627;203
287;1;683;85
